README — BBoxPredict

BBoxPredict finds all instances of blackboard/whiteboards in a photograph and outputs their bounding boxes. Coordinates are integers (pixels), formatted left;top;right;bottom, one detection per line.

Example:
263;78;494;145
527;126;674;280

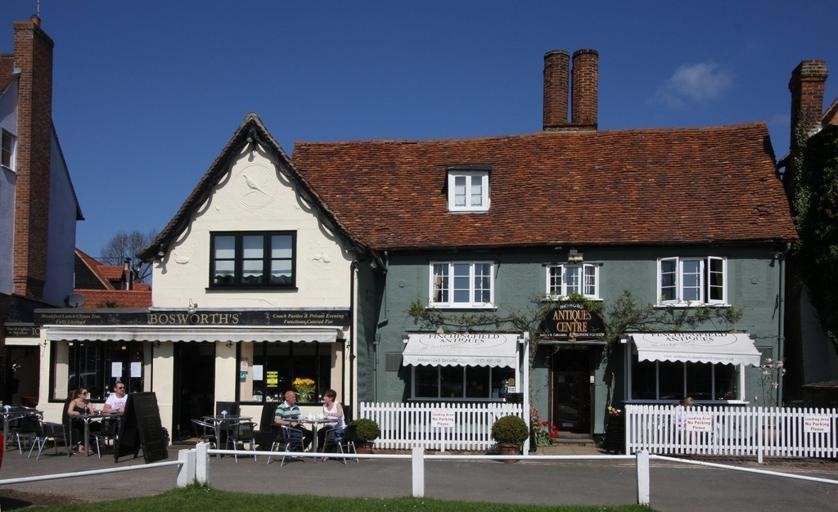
132;392;168;464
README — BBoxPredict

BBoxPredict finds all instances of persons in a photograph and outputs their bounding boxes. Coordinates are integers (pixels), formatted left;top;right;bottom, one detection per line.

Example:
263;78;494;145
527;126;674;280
273;390;314;463
66;387;98;454
670;396;694;426
315;388;348;460
102;380;129;414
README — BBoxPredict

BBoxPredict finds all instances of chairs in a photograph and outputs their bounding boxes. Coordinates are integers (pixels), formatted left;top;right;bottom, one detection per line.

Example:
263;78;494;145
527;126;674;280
177;402;358;465
1;402;170;464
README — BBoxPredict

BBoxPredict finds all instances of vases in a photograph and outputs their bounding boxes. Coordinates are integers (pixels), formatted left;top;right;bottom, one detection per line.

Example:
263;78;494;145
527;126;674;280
298;391;309;402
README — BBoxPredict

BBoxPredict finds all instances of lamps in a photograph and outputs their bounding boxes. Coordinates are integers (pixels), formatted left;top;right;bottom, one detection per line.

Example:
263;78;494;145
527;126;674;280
188;297;198;314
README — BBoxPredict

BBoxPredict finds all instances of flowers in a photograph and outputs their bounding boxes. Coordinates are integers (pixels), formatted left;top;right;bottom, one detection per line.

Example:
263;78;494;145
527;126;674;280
292;377;316;399
529;407;559;438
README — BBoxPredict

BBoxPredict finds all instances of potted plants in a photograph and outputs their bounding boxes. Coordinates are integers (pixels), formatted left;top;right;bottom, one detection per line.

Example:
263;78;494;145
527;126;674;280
491;416;528;463
344;418;380;458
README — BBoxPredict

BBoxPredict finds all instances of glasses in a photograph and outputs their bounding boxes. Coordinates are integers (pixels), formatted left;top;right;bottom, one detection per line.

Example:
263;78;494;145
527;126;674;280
80;391;88;394
120;386;126;389
